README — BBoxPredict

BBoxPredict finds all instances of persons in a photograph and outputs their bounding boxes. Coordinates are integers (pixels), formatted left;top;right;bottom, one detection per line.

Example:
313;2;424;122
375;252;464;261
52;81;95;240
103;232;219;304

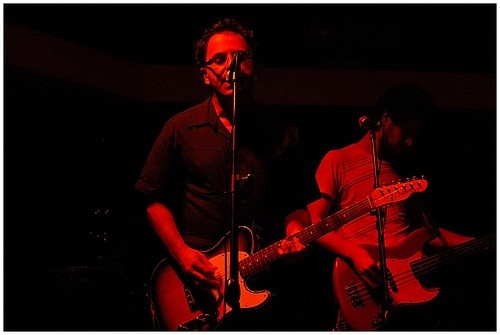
137;19;313;331
278;82;479;331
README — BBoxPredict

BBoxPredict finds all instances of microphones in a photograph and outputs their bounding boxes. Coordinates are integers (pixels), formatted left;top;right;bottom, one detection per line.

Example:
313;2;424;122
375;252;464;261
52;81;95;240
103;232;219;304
225;53;239;84
358;115;382;129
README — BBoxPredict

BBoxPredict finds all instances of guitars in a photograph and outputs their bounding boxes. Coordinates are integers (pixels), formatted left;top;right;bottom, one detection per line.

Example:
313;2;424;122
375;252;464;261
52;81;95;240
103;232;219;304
150;174;430;331
333;225;496;331
